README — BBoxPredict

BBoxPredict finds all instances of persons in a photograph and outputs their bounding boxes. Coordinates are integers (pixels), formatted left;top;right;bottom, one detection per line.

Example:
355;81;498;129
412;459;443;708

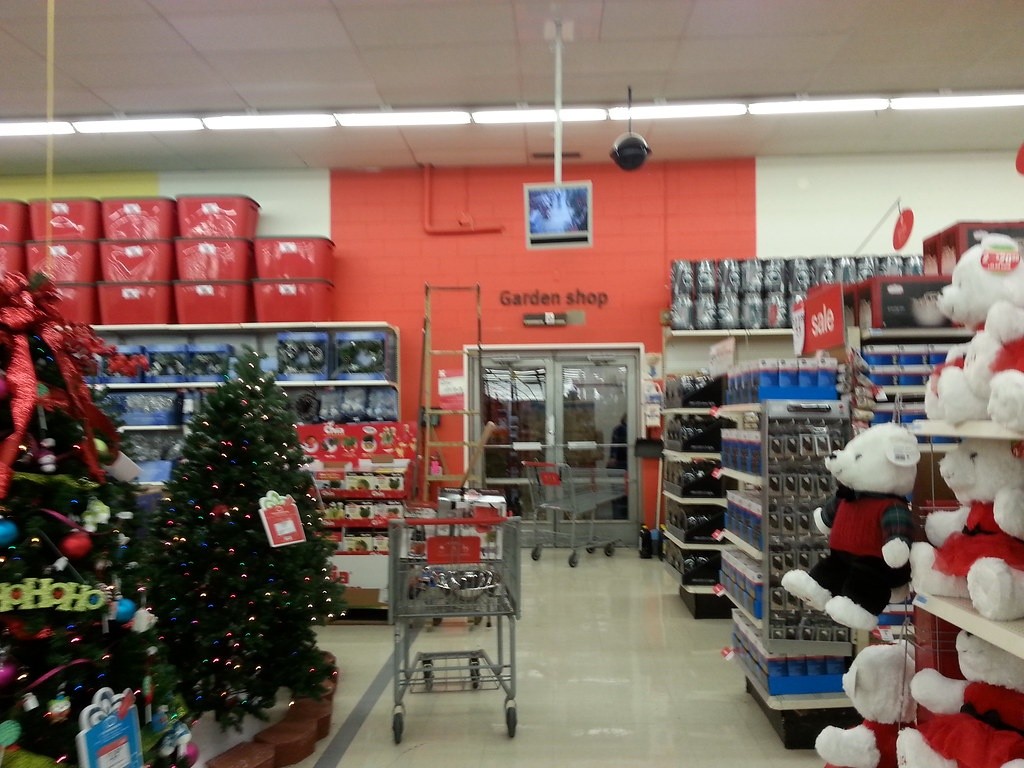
605;415;627;520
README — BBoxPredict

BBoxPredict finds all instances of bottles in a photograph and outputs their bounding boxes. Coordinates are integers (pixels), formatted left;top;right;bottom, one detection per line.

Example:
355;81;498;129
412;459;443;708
639;526;651;559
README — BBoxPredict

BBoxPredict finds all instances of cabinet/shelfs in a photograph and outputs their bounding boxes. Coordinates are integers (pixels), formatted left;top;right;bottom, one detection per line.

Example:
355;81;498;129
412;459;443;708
55;320;417;622
656;329;1024;768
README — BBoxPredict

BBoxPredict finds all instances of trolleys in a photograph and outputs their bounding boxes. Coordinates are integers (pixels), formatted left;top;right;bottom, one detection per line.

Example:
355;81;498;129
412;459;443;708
386;503;522;744
522;460;638;568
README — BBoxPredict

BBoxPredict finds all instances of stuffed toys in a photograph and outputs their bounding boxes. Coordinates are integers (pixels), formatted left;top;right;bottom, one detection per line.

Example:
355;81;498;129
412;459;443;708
896;630;1024;768
919;233;1024;432
908;439;1023;622
815;638;914;768
779;423;921;632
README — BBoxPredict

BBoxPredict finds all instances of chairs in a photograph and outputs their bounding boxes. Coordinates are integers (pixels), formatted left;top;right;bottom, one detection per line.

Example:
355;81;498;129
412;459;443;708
841;219;1024;328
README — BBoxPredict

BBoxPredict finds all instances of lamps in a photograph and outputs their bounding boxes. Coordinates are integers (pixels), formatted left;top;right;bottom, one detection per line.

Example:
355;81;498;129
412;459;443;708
0;86;1024;136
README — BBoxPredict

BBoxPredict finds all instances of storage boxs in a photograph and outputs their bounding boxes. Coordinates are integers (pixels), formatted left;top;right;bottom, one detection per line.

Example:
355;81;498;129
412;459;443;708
174;239;254;281
438;486;507;559
335;331;394;380
173;280;249;324
43;283;94;326
98;238;176;283
253;235;335;284
142;344;187;383
175;193;260;242
184;344;233;382
99;196;176;241
250;279;334;321
28;197;104;240
274;332;333;382
101;346;144;382
0;199;31;274
23;239;99;283
862;345;899;366
96;282;174;324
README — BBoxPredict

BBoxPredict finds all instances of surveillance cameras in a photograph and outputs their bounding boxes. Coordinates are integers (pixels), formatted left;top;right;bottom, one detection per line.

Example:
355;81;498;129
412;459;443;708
609;131;652;172
